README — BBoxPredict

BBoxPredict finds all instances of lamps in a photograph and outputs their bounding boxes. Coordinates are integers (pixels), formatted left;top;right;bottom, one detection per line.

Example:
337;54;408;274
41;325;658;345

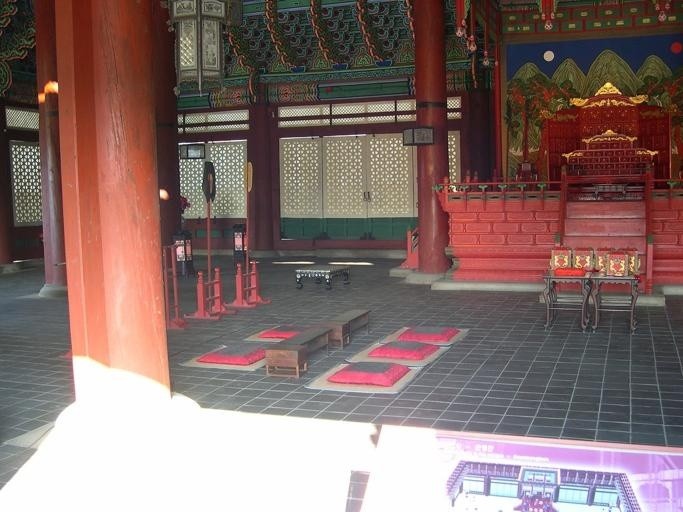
232;223;249;268
172;230;195;279
160;0;231;101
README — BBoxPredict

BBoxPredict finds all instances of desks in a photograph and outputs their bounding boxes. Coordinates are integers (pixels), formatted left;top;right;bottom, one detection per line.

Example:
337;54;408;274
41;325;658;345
263;309;371;379
294;264;351;290
539;267;641;338
592;183;629;200
